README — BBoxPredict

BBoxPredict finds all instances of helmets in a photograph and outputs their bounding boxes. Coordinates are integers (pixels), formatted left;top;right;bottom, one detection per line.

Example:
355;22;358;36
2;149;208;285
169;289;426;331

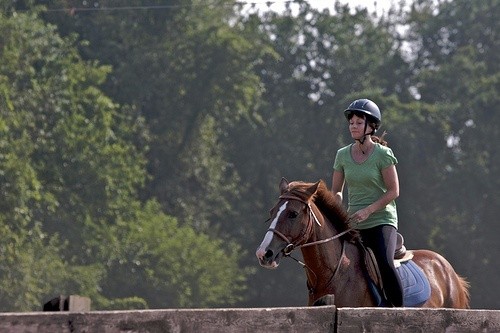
344;98;381;124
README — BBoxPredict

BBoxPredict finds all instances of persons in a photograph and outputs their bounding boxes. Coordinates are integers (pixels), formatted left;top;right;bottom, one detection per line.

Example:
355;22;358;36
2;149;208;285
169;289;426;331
330;98;404;307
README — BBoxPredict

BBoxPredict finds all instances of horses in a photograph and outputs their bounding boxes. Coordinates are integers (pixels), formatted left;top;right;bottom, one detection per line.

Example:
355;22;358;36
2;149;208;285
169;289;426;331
255;176;472;309
